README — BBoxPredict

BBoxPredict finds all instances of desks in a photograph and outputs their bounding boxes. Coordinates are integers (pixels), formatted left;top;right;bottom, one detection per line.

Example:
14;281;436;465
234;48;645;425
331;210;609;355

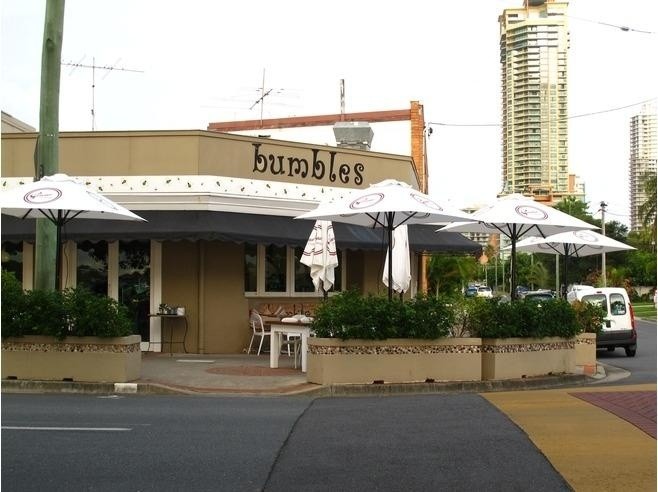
264;321;317;373
145;314;189;356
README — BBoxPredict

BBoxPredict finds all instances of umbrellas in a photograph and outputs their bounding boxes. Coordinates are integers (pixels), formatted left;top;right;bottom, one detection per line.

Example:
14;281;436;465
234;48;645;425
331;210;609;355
381;224;411;293
500;228;638;294
436;191;603;304
297;190;337;296
292;179;481;307
1;173;149;292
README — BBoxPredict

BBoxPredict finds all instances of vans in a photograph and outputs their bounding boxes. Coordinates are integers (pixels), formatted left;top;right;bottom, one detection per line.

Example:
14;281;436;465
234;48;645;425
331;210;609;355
567;288;637;356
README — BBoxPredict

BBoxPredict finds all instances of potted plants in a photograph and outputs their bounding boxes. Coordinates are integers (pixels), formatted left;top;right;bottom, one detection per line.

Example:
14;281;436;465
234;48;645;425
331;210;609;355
159;303;186;316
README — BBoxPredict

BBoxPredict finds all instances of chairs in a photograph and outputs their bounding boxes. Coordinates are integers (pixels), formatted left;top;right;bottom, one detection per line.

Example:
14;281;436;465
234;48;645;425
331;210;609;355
247;308;271;356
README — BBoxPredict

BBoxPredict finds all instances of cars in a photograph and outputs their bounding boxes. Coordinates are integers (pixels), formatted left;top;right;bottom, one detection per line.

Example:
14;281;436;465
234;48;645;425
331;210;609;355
495;285;594;309
466;283;493;299
